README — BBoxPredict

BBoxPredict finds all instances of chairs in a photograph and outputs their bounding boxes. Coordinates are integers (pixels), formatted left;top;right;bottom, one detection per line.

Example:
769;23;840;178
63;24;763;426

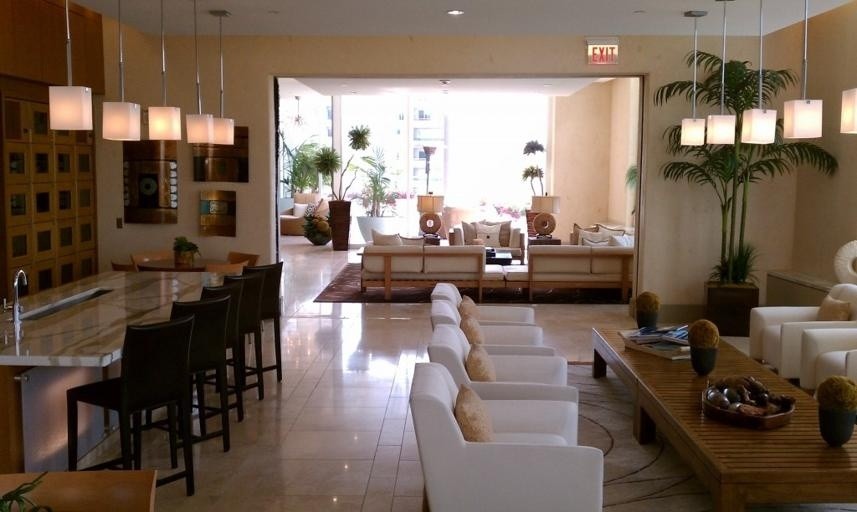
130;295;232;467
106;250;261;277
411;362;606;512
187;264;283;426
429;298;557;358
751;282;857;380
429;322;579;401
798;328;857;396
280;190;317;236
62;314;198;498
429;281;544;346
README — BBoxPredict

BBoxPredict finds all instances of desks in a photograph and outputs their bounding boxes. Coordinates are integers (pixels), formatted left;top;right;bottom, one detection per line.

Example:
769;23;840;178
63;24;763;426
528;236;561;244
1;468;158;512
423;238;440;245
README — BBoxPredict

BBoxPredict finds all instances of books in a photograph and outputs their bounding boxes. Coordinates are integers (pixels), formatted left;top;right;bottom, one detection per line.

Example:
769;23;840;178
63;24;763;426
617;316;691;364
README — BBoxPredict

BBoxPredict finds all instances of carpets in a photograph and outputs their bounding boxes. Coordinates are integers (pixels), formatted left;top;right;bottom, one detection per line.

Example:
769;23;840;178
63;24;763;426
314;261;632;304
564;361;856;512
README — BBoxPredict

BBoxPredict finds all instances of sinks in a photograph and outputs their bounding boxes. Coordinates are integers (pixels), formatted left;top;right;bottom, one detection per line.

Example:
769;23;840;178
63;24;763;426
9;287;115;322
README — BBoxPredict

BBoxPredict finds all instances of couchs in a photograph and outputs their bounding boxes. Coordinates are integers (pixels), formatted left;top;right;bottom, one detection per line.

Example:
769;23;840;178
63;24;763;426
448;225;526;265
503;244;634;302
356;244;506;303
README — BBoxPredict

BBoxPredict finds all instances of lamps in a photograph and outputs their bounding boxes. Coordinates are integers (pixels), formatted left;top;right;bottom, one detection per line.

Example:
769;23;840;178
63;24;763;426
417;191;444;238
185;0;215;145
531;195;560;238
706;1;736;143
679;10;706;147
146;0;183;142
840;85;857;135
208;8;236;146
740;0;778;144
782;0;824;139
101;0;142;143
47;0;94;131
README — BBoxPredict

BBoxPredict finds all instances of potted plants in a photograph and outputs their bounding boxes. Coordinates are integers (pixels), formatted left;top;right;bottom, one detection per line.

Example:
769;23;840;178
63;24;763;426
816;379;857;446
633;289;660;334
354;150;400;243
686;317;719;375
172;236;198;265
523;140;547;235
651;49;839;333
316;124;371;251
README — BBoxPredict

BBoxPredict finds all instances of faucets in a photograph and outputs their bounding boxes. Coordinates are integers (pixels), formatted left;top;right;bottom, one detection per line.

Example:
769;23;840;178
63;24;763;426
11;269;28;326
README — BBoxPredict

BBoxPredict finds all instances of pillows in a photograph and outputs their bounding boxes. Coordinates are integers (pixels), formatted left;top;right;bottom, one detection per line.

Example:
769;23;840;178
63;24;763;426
570;221;635;247
460;316;486;349
461;293;484;321
464;343;499;384
817;293;851;322
487;220;512;247
476;222;502;246
462;220;486;245
453;383;494;445
371;227;403;245
293;202;316;216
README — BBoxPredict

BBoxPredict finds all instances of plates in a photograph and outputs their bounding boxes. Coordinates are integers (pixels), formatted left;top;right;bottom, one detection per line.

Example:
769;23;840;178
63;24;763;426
701;386;795;430
